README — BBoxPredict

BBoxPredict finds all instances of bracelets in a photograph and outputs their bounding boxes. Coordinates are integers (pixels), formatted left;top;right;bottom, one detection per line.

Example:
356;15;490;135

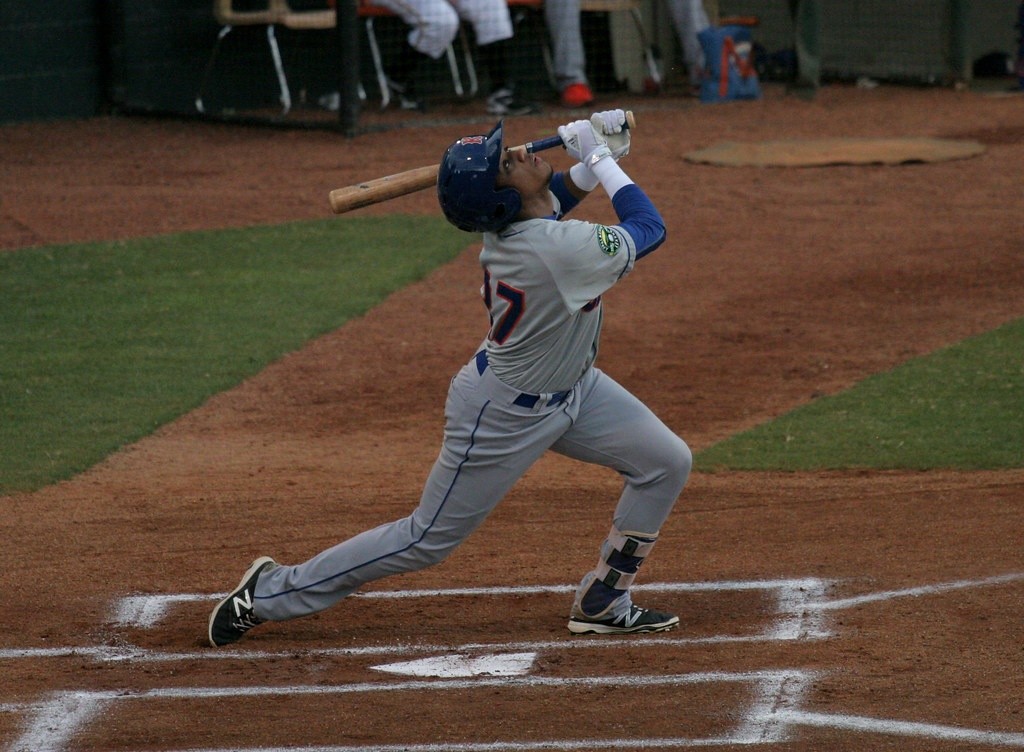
589;152;612;170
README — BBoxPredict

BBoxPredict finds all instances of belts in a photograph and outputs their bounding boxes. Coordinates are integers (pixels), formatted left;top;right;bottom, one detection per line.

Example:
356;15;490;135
476;350;569;409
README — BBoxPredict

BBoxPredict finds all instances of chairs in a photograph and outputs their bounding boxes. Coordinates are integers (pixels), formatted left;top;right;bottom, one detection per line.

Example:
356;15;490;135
191;0;741;125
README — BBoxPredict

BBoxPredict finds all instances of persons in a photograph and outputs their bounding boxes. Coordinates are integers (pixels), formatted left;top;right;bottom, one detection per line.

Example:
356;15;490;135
545;0;710;107
208;108;692;647
377;0;541;116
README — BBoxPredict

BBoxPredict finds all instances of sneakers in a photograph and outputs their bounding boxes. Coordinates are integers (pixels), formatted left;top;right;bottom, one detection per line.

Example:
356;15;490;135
208;554;277;648
486;93;541;118
562;84;593;109
567;601;680;634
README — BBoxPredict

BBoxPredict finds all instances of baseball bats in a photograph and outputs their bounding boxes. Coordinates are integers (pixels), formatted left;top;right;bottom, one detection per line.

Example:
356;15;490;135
324;109;638;217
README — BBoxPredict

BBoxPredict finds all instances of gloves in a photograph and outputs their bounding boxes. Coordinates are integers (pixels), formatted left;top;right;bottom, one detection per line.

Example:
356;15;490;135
591;109;631;160
557;119;613;169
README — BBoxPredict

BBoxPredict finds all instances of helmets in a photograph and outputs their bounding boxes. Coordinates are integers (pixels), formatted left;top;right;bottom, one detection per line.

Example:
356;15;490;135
436;119;522;233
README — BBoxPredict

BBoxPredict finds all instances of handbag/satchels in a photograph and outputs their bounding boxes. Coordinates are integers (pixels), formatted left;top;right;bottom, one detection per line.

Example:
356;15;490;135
695;24;764;102
